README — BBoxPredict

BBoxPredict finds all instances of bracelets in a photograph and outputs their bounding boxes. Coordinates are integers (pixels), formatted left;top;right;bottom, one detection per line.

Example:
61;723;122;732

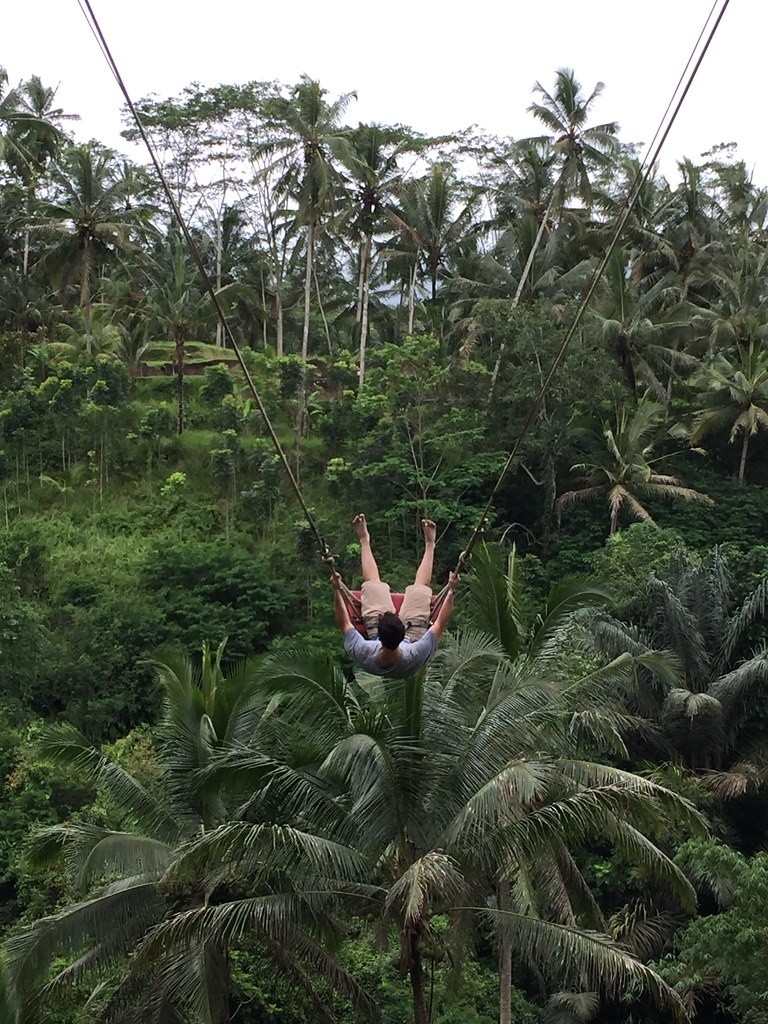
448;591;456;595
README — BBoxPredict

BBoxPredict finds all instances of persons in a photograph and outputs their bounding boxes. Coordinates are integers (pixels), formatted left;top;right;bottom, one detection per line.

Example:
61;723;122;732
329;513;458;678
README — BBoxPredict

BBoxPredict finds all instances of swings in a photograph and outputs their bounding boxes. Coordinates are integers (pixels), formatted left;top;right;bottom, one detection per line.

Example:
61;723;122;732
83;0;729;642
